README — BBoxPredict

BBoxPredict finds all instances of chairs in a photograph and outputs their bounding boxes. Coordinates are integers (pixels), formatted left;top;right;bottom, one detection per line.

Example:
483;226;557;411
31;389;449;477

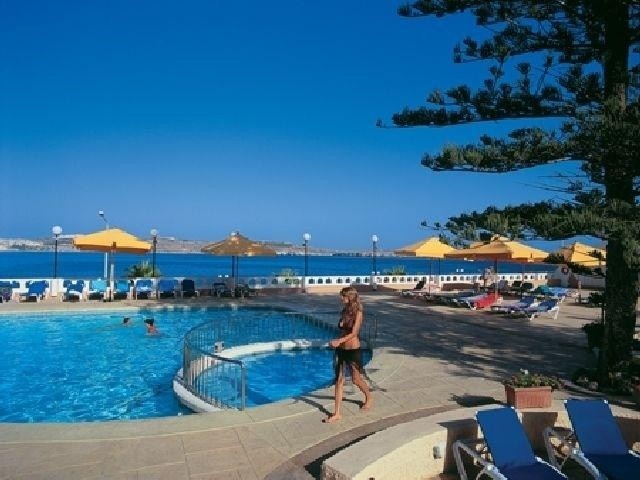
542;398;640;480
0;278;262;304
397;280;579;322
450;406;573;480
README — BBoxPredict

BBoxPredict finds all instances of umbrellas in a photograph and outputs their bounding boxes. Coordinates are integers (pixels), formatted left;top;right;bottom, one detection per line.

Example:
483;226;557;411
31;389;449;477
73;228;151;301
202;231;276;286
394;233;607;299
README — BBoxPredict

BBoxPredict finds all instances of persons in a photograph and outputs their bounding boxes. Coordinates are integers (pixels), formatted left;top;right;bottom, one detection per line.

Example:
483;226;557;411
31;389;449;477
124;318;131;325
144;319;158;335
322;287;372;422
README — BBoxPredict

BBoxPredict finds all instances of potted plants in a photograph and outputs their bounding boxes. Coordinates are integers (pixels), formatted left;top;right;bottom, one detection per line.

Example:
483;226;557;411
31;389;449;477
500;367;564;407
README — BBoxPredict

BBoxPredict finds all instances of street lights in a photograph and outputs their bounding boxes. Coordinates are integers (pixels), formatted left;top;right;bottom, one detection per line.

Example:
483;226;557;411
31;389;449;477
51;225;62;277
229;231;237;278
151;228;158;276
371;234;379;275
98;209;110;279
303;232;311;275
